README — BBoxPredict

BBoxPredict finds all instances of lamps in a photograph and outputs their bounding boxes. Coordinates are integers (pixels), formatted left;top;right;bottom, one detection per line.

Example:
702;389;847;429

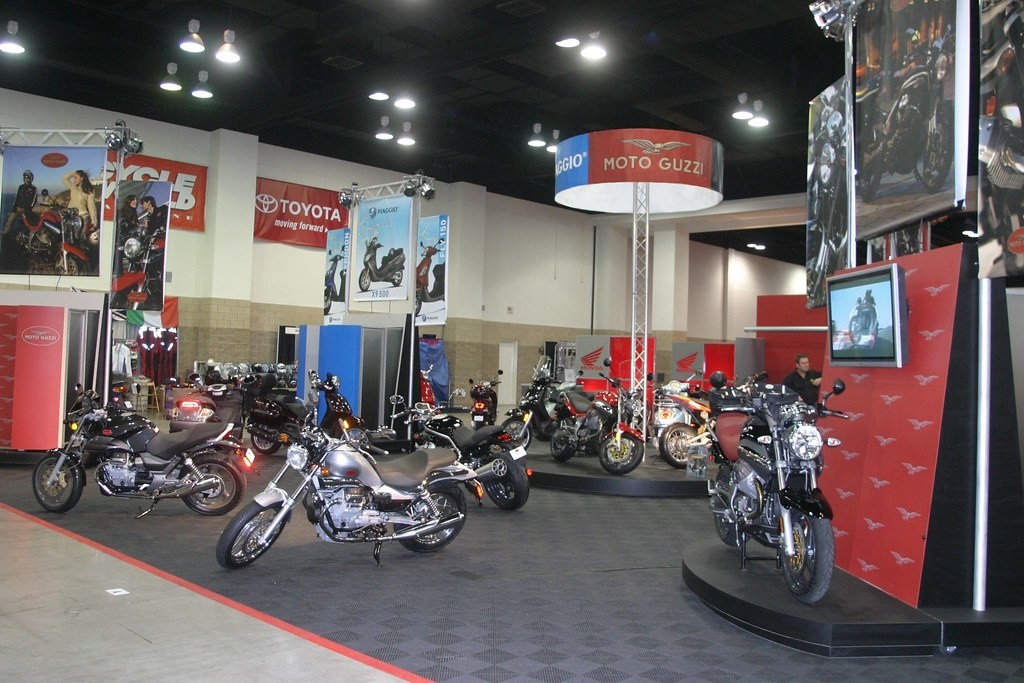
189;70;213;99
105;126;143;156
368;86;389;100
547;130;560;151
733;92;754;119
405;171;436;200
160;63;182;92
748;101;769;127
581;29;607;61
179;20;205;54
215;30;240;63
397;121;416;145
527;123;546;147
0;20;25;53
338;186;353;208
393;91;416;108
374;116;393;140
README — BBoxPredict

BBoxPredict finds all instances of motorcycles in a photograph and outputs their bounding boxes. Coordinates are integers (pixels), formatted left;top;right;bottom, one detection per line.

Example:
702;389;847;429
12;188;90;276
214;392;484;572
32;383;255;521
806;74;850;307
109;223;167;310
166;360;847;603
853;21;955;204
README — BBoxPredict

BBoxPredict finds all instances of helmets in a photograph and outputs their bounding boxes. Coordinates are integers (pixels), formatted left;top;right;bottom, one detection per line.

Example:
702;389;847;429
288;364;297;374
89;231;98;244
24;170;34;180
208;370;223;381
224;365;234;375
267;365;274;372
290;380;296;388
277;364;286;373
252;364;262;371
709;370;727;388
238;363;248;373
243;375;255;383
41;189;50;196
278;380;287;388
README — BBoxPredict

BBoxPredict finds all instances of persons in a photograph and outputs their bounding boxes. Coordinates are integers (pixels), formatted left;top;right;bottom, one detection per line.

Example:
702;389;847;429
138;196;169;278
3;170;37;235
120;194;142;241
63;169;99;240
862;289;875;305
783;353;823;408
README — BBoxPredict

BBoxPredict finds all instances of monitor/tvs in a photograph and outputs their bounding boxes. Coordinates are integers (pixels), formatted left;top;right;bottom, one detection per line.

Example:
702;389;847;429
826;263;909;368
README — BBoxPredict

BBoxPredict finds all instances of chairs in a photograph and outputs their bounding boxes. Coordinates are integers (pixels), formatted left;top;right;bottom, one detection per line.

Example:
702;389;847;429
135;378;160;413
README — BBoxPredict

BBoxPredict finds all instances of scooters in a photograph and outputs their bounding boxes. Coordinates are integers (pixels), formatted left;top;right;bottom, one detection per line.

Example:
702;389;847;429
358;236;406;292
415;238;445;316
323;245;347;314
